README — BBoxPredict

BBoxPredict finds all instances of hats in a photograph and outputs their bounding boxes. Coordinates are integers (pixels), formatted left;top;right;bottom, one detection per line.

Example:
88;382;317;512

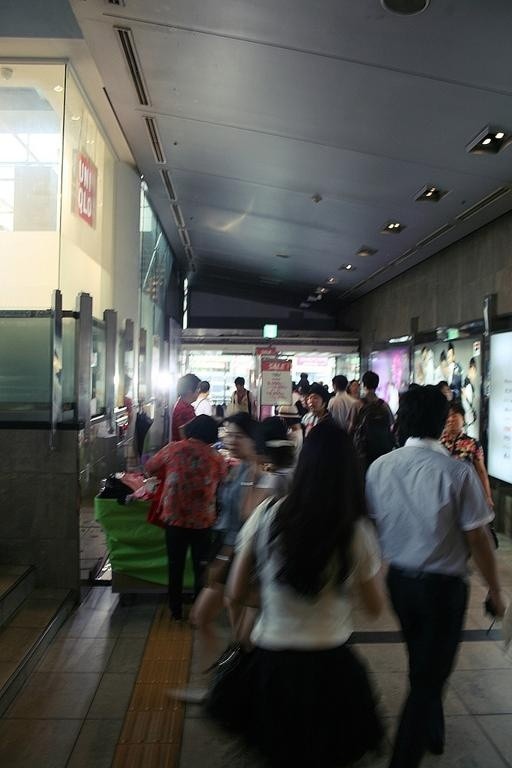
276;405;302;418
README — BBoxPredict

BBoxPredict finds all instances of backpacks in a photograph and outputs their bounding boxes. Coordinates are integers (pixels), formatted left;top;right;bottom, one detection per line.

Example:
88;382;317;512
355;398;393;463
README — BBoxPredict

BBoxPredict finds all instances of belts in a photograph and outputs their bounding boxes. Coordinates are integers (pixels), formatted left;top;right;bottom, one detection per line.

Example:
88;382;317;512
387;569;458;582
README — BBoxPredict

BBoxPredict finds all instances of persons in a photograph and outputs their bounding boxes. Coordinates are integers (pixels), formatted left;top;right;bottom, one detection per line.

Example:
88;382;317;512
432;350;449;385
201;416;384;766
440;399;495;512
446;344;462;410
187;412;263;666
462;358;477;405
240;417;294;646
146;413;227;619
330;374;357;430
121;376;133;437
439;382;453;403
414;346;434;383
275;371;333;467
172;374;201;440
346;371;398;477
192;380;213;417
227;377;258;420
367;383;506;767
212;403;229;427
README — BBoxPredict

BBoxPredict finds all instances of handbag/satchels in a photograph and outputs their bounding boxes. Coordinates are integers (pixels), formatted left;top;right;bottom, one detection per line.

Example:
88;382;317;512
147;470;166;530
204;639;244;719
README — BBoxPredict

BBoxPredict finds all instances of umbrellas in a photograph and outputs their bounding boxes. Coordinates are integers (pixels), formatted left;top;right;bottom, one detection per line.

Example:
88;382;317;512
136;401;152;460
